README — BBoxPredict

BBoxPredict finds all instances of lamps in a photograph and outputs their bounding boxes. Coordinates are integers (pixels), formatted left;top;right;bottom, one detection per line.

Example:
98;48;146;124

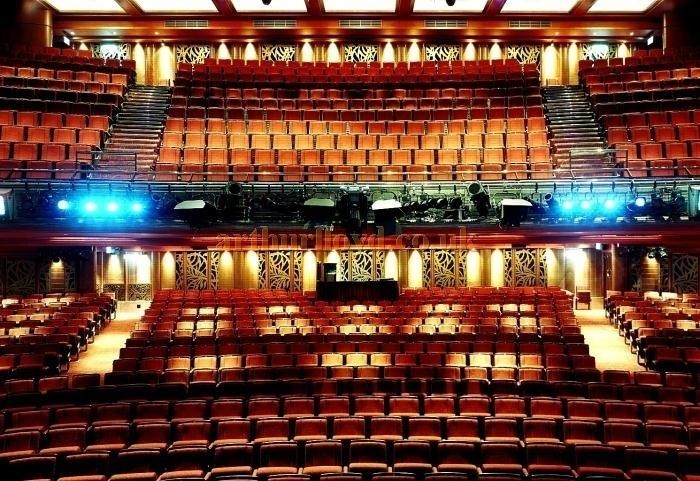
158;189;698;219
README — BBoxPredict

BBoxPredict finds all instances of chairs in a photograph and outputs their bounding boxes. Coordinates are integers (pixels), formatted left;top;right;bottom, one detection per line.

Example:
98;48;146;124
0;35;699;186
0;285;699;481
